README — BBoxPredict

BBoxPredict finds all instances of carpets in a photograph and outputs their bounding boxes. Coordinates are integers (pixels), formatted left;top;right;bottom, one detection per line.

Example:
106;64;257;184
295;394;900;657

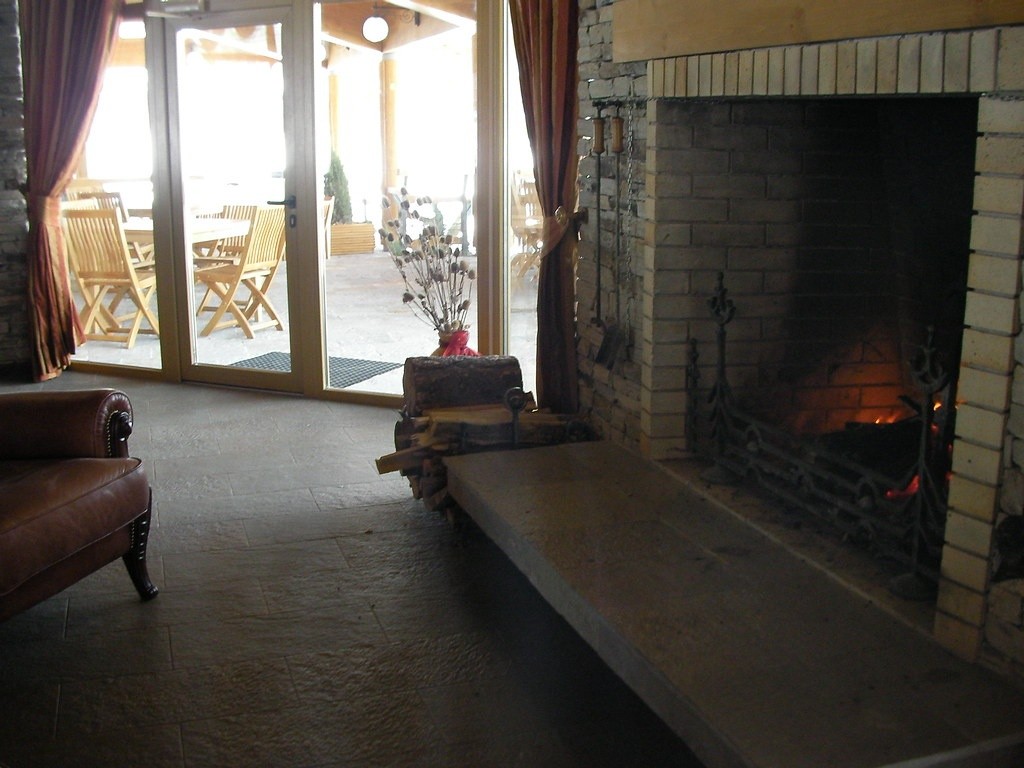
227;352;405;389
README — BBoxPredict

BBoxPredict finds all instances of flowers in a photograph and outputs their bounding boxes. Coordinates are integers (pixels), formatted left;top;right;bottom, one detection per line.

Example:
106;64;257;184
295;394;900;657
378;186;477;333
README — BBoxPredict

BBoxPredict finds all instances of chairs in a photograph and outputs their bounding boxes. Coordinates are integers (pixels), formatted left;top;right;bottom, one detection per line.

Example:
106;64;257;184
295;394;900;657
59;180;286;350
323;196;335;259
509;174;545;279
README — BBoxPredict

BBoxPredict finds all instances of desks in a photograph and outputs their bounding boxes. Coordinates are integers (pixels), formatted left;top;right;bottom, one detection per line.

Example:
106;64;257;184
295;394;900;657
122;217;251;261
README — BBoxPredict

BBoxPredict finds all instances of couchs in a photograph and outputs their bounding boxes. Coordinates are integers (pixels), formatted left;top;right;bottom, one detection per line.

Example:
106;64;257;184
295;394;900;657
0;388;158;627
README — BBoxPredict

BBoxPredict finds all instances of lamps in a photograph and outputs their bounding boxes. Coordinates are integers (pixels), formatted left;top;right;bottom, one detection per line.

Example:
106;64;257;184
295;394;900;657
362;2;421;43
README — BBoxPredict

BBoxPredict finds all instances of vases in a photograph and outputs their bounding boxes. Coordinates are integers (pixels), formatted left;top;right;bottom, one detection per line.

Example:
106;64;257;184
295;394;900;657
431;331;477;357
